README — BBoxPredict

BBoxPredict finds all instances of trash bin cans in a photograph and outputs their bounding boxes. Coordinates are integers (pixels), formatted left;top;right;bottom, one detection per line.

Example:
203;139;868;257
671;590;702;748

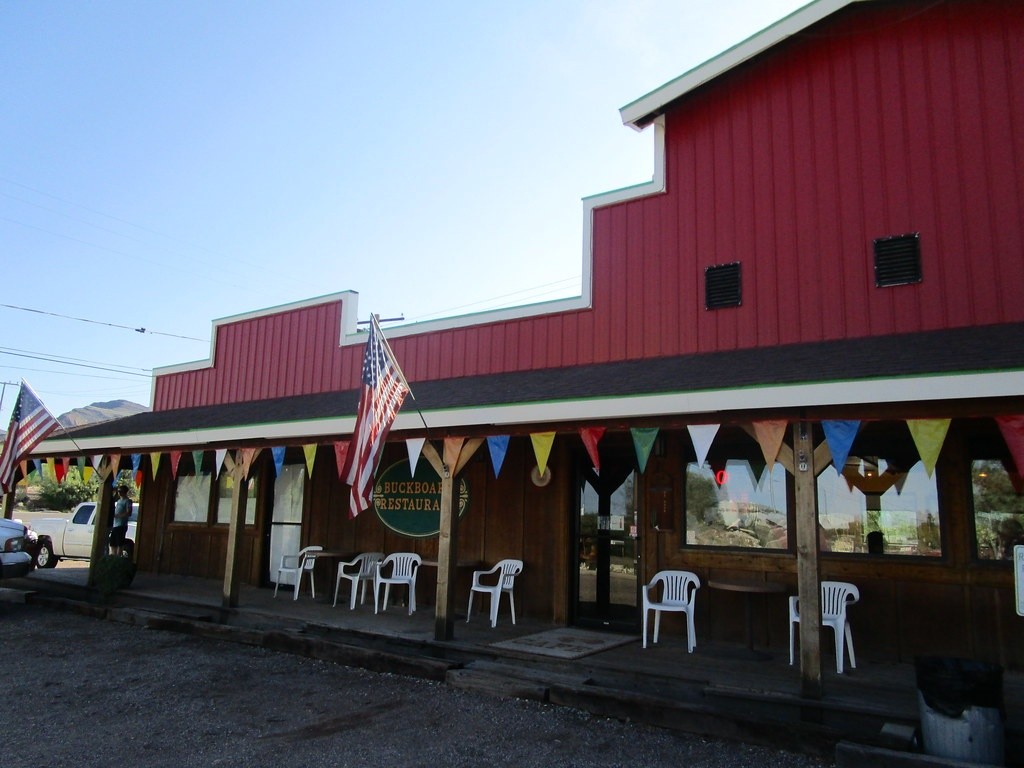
915;655;1007;765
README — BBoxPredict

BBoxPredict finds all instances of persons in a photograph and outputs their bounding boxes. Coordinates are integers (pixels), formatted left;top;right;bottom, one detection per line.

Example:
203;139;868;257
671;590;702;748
108;485;133;555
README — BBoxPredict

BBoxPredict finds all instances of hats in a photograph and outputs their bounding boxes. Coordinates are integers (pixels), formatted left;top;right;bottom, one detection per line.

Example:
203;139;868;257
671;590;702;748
990;518;1024;545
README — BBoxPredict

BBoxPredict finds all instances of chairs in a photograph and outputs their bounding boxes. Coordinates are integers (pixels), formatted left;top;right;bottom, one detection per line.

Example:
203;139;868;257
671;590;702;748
642;571;700;653
332;553;384;609
789;581;859;674
274;546;323;601
371;553;422;616
466;559;523;628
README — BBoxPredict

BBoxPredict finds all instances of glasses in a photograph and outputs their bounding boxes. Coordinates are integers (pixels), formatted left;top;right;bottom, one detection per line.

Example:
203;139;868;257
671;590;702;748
117;490;122;492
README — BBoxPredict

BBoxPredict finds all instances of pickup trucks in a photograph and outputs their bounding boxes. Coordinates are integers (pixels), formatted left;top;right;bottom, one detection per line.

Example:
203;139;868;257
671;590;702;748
28;502;139;571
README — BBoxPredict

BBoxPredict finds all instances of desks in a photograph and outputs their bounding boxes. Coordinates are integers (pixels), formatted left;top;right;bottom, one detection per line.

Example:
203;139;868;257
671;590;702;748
315;550;356;604
421;558;468;609
706;575;784;661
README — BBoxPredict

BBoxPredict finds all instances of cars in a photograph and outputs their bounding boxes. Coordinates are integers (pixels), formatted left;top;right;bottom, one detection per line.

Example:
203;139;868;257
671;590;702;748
0;519;39;580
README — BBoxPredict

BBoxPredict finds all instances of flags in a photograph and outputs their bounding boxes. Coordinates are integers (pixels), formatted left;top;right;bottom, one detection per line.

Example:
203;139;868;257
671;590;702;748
338;316;409;519
0;381;60;494
